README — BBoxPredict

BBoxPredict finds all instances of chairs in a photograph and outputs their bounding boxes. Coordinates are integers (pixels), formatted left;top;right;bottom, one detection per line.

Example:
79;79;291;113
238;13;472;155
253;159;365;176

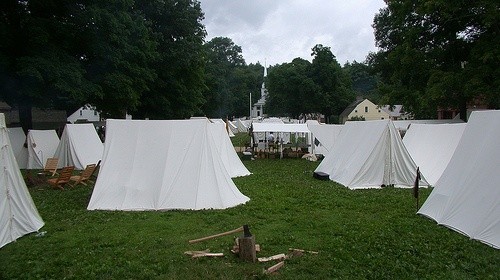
36;158;96;191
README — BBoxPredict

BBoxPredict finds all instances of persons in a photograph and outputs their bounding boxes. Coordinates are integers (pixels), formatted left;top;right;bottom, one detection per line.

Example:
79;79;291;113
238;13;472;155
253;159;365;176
268;132;275;152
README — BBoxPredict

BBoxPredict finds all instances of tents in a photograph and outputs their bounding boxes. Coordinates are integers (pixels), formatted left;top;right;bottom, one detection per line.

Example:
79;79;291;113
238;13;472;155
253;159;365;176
0;110;500;250
